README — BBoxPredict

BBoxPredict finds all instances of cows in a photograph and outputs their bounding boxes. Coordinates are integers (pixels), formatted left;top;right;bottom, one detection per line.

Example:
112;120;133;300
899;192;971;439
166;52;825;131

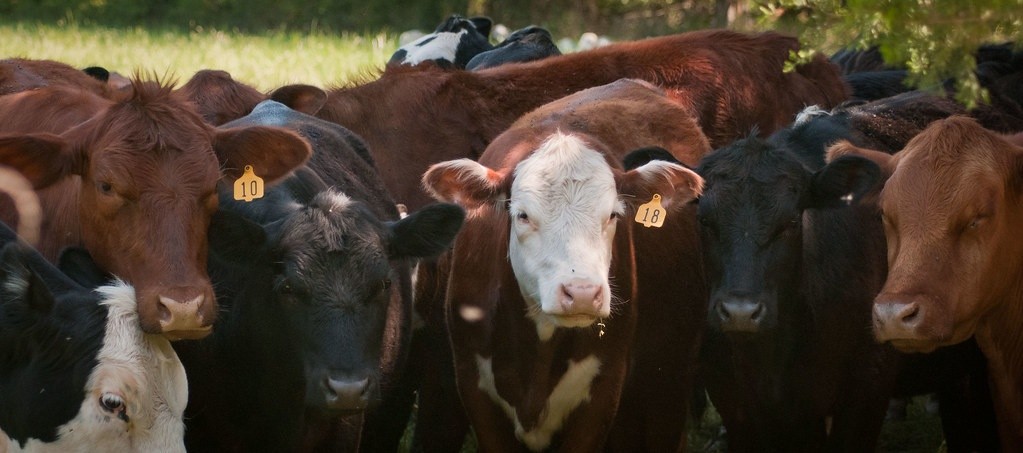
0;11;1023;453
